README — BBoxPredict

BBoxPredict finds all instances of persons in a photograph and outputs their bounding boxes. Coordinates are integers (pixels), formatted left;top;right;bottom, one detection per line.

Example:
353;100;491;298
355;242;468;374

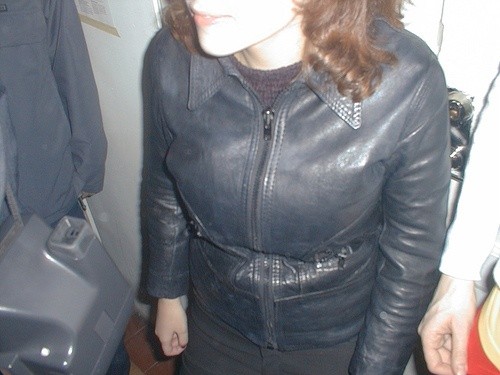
416;63;500;375
139;0;453;375
0;0;131;375
